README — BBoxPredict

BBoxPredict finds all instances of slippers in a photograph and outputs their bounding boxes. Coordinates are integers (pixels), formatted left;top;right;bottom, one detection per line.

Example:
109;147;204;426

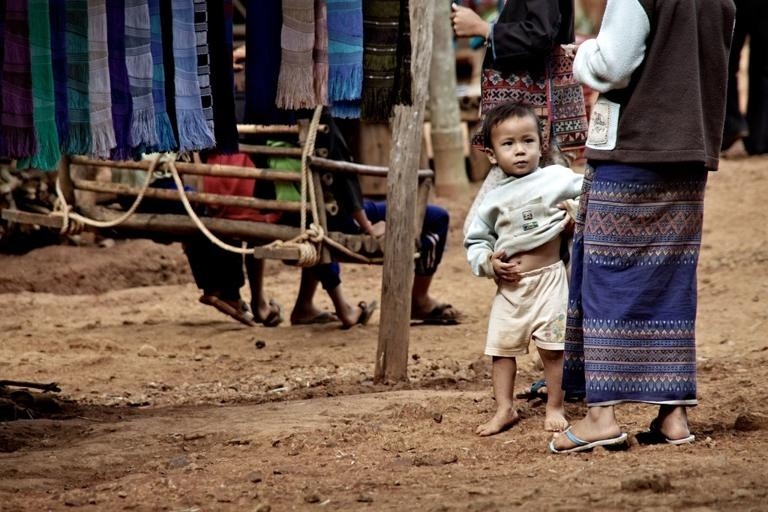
209;297;258;328
291;311;341;325
410;303;464;324
651;418;695;445
265;298;284;328
550;427;627;454
358;300;376;325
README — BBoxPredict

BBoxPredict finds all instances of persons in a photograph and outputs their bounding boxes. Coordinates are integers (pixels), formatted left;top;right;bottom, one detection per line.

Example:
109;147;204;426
300;112;462;327
117;173;255;325
200;151;377;330
451;0;594;401
547;0;741;455
201;147;283;325
461;99;585;436
